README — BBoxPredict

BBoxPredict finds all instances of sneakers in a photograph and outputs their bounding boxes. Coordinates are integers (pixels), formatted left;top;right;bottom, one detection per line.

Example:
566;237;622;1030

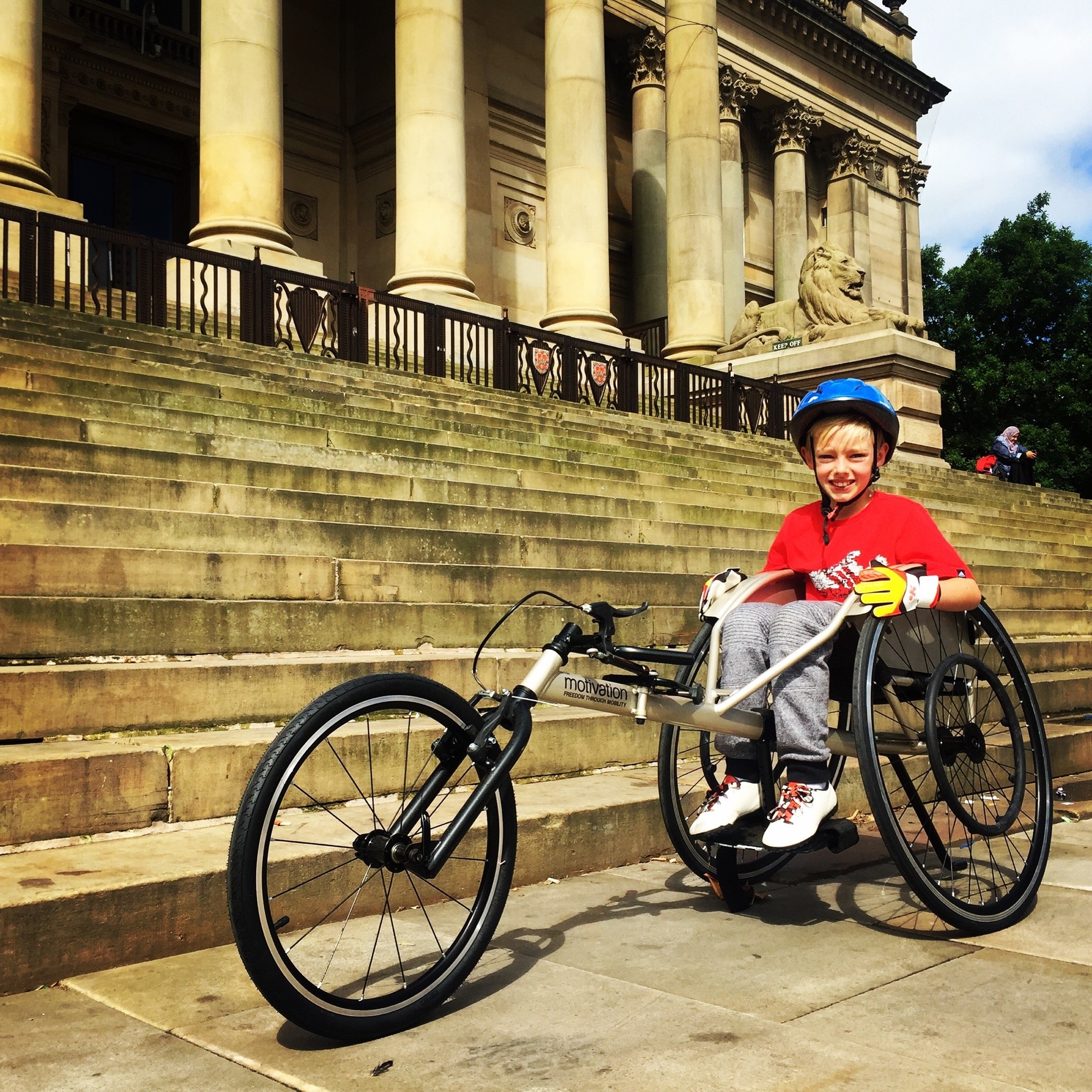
761;781;838;853
689;774;765;837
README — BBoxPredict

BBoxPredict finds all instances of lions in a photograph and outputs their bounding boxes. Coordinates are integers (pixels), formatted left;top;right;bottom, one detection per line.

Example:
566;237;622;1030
716;242;926;354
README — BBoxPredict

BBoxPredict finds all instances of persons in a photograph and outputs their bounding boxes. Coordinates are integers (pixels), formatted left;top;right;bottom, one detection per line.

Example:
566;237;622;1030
992;426;1037;486
690;377;982;848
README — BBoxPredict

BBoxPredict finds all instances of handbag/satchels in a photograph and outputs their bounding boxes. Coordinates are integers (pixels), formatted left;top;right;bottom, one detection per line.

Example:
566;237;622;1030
993;459;1009;482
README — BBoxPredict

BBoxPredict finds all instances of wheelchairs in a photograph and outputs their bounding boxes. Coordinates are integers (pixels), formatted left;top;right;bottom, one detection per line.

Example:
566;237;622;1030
222;570;1058;1042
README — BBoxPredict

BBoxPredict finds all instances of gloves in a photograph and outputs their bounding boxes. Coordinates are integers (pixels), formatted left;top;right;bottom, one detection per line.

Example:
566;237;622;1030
698;567;748;622
854;565;941;620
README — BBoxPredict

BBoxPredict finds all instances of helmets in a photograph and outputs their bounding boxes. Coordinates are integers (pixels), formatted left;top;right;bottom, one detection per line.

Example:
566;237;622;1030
789;378;900;465
